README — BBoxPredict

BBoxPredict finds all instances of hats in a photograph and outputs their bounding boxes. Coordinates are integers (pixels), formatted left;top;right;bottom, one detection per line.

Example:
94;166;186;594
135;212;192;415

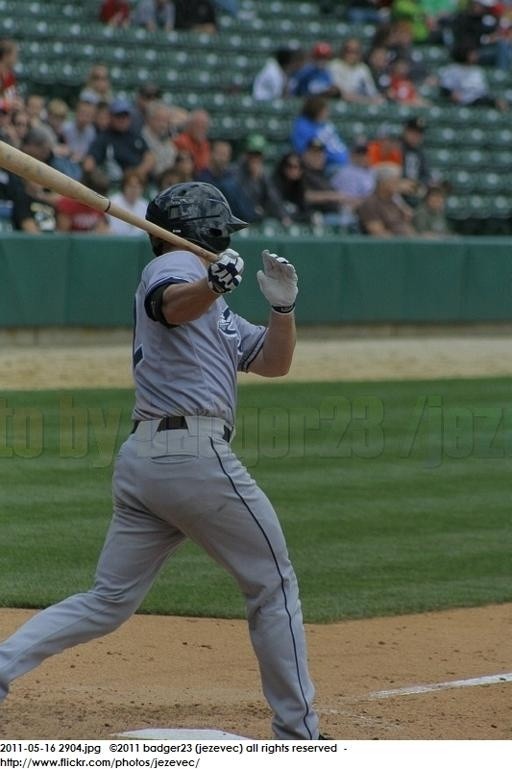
408;119;427;134
111;101;134;116
307;139;327;152
313;42;333;57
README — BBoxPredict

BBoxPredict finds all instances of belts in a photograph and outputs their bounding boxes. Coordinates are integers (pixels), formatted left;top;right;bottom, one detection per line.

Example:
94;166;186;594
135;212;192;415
131;419;231;442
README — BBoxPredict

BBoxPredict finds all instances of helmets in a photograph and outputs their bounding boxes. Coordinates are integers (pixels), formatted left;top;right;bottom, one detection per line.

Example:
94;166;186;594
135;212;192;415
146;182;250;253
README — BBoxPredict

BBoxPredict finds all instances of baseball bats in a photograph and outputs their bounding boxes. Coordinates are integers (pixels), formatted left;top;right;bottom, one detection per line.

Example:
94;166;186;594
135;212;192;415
1;142;218;262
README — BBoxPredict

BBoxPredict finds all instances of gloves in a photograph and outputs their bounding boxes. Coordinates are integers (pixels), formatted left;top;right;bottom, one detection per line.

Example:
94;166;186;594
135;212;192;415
207;250;245;295
257;249;299;315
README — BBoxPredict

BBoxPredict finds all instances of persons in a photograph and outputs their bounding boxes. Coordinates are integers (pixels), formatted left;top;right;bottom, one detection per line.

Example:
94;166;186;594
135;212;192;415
0;181;328;739
2;1;512;236
